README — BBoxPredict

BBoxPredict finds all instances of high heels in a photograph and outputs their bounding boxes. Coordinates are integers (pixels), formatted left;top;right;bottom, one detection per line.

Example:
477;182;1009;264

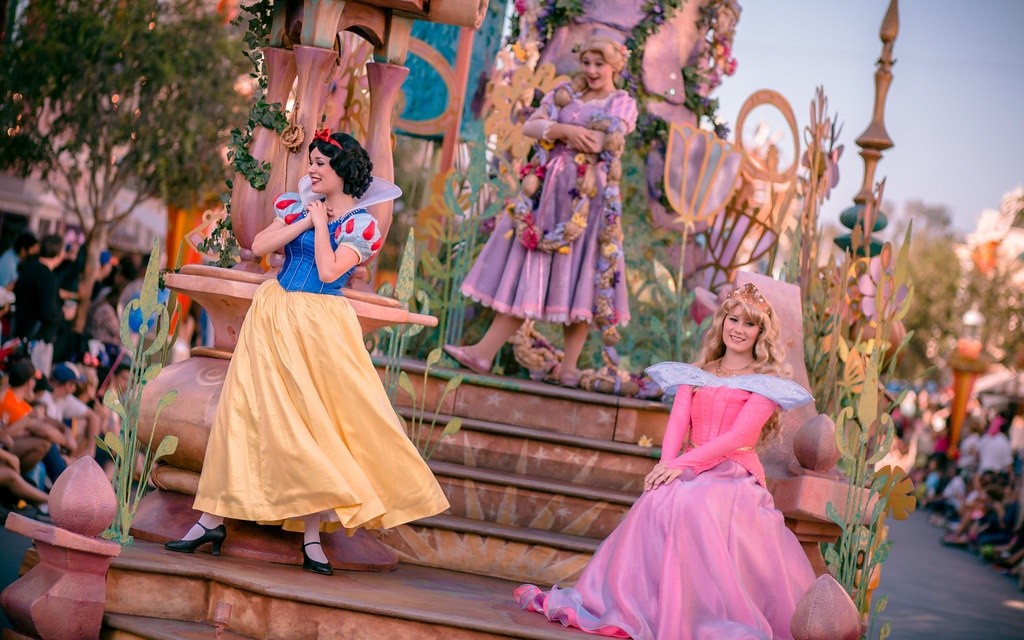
302;542;332;575
165;523;226;555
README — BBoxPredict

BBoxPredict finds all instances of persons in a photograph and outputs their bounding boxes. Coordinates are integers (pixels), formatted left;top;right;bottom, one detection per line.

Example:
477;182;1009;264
443;38;639;387
164;133;449;576
513;282;813;639
877;387;1023;591
1;229;216;525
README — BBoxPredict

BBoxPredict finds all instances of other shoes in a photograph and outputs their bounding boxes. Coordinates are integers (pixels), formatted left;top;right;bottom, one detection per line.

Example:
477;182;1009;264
929;512;1024;586
443;345;492;375
560;370;579;388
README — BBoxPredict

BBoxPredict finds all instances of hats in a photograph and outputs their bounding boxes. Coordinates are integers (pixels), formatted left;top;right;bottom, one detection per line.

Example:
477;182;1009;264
8;359;44;387
54;362;86;383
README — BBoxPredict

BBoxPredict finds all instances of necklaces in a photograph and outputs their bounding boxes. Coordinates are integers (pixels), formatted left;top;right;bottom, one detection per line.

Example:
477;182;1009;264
715;358;756;380
